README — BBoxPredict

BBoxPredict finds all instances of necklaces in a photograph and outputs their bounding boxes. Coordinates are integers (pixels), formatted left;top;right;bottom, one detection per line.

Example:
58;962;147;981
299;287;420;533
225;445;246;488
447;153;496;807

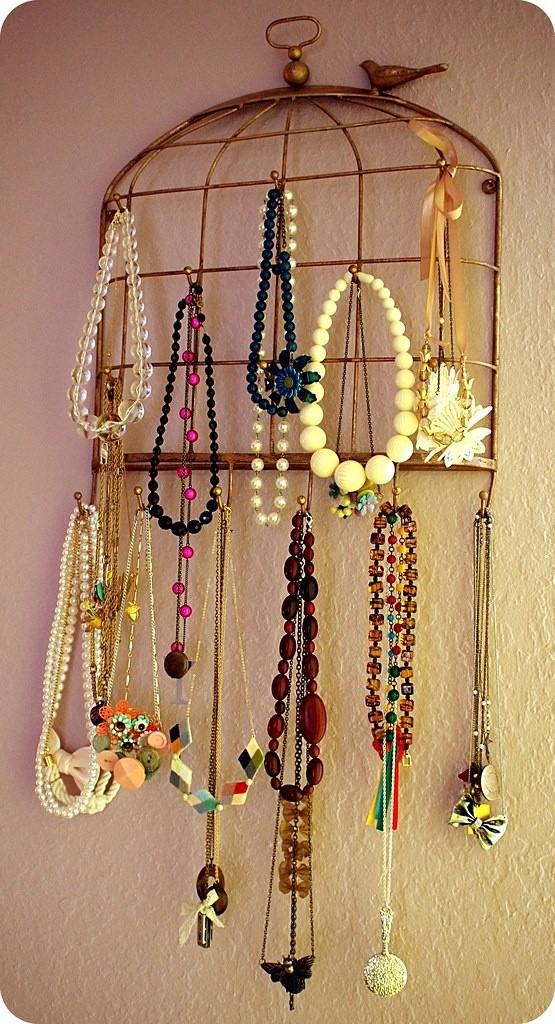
32;123;507;1011
